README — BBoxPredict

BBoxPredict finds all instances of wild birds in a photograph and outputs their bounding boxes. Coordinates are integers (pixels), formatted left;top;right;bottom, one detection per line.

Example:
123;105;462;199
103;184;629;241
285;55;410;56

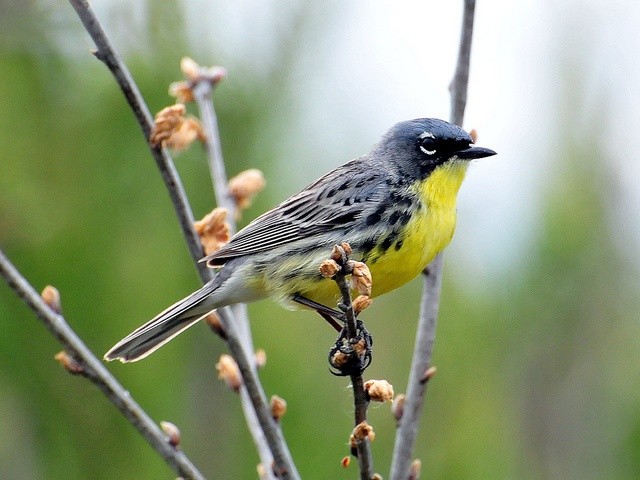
102;117;499;377
